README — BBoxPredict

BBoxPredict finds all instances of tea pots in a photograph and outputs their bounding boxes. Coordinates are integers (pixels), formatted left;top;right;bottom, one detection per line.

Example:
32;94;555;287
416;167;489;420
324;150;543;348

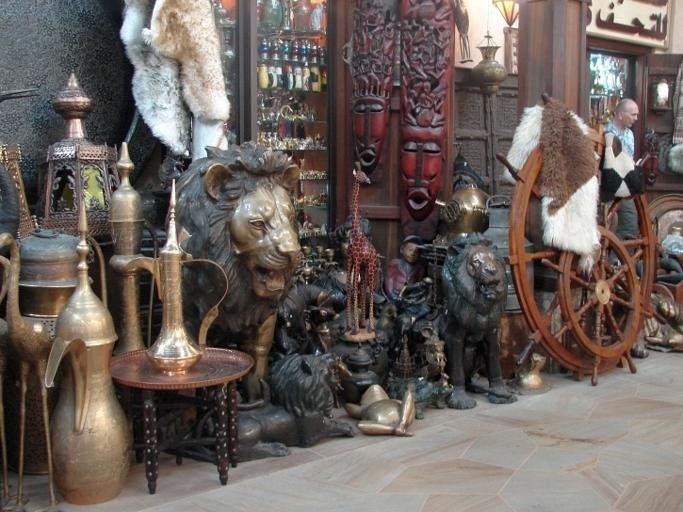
119;177;229;377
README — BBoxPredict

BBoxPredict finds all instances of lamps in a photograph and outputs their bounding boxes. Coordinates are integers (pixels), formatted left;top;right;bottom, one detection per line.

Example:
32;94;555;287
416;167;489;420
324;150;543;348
494;0;520;28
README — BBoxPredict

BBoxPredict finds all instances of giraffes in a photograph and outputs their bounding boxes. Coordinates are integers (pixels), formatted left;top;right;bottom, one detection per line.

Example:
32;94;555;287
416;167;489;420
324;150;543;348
345;170;377;334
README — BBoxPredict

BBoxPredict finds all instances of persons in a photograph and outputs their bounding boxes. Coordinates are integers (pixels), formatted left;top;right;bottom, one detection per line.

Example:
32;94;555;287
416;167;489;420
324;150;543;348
387;235;427;300
602;97;639;234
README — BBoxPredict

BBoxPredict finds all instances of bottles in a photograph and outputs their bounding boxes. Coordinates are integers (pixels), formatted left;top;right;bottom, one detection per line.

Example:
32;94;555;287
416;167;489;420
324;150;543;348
258;36;326;64
661;226;682;256
255;131;280;151
256;63;322;92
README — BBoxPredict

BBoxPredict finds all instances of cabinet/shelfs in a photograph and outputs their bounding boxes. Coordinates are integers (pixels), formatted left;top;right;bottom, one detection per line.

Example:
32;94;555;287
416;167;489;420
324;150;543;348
188;1;332;274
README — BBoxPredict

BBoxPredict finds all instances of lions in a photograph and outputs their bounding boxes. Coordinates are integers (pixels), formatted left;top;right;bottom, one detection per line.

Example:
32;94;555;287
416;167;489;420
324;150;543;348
165;140;303;411
440;244;517;410
